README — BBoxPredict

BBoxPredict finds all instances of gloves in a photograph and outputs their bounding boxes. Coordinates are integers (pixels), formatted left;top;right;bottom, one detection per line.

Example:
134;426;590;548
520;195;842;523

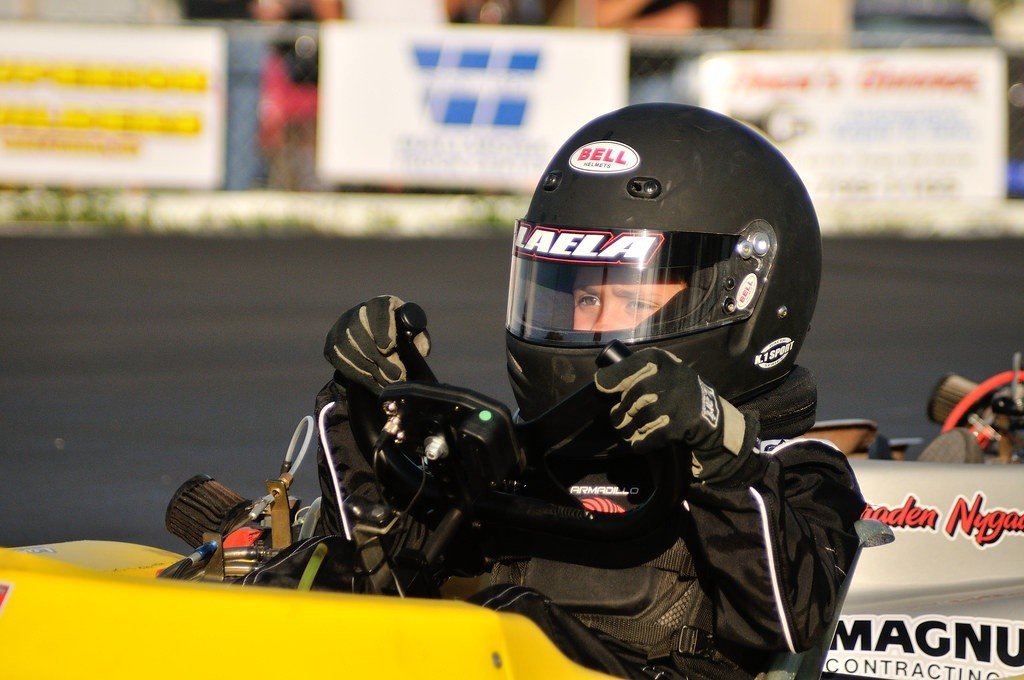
595;347;760;484
323;296;432;394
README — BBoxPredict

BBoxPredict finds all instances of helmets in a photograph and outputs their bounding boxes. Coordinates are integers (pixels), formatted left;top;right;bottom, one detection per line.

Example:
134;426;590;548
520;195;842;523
505;102;821;479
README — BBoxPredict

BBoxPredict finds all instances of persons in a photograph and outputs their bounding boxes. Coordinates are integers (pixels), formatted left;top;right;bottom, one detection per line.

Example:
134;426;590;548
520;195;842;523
314;103;867;679
